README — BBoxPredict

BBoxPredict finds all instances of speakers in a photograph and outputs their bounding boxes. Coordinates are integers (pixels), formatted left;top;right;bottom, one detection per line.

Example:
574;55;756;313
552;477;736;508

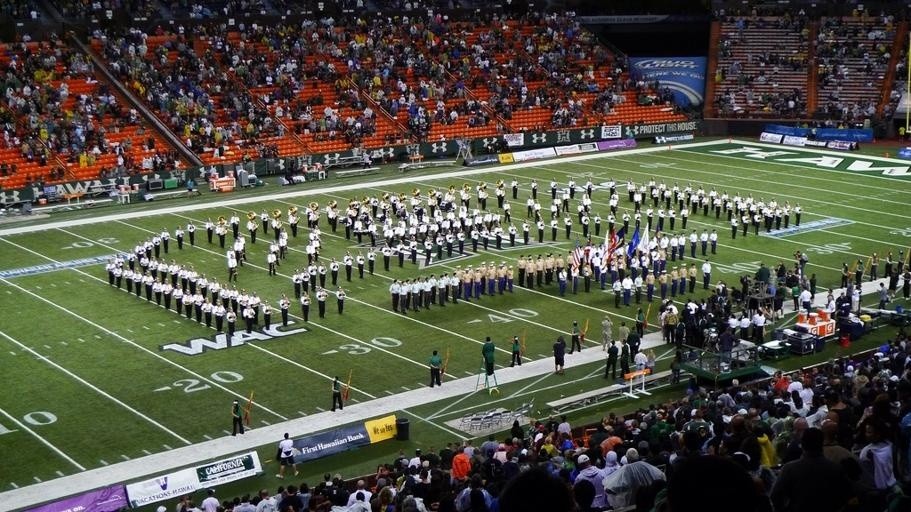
276;443;282;461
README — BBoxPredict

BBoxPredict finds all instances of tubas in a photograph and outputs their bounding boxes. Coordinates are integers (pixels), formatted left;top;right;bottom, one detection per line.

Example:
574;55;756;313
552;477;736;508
448;177;504;197
216;198;338;226
346;185;438;207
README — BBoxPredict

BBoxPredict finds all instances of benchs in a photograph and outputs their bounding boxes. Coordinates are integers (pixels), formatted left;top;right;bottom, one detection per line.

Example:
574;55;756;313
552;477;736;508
546;368;687;413
713;15;898;118
1;21;689;188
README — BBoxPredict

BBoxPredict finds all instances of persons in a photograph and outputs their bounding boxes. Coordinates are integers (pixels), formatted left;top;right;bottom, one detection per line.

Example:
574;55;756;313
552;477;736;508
330;376;343;412
231;400;244;436
460;248;910;512
156;439;472;511
1;1;911;200
430;350;442;388
106;177;801;334
276;433;299;479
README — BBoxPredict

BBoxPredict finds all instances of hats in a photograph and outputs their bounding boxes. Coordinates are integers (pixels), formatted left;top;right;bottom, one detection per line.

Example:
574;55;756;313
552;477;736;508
606;451;617;466
578;454;590;463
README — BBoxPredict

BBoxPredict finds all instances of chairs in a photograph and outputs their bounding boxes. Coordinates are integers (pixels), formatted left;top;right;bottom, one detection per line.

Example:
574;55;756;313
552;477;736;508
458;398;535;434
